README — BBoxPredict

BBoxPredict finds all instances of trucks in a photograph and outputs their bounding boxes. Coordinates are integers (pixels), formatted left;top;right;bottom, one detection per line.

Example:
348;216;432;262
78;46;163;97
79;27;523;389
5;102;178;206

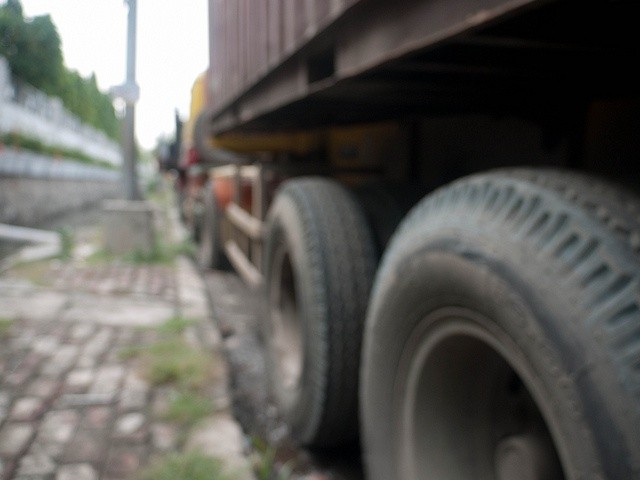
150;1;639;480
